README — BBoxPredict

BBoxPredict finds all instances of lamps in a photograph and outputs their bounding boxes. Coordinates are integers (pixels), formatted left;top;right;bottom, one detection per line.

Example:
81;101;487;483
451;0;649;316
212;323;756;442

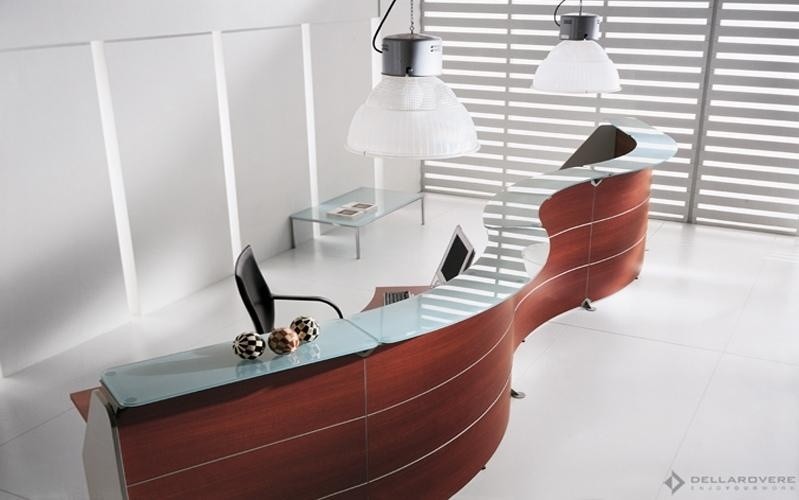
343;0;482;160
530;0;622;94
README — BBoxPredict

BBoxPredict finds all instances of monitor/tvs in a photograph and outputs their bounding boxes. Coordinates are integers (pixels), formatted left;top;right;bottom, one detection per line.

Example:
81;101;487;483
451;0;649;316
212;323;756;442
430;225;476;283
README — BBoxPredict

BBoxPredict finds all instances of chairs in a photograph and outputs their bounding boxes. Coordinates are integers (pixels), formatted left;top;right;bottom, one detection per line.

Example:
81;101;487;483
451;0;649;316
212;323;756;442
233;244;343;334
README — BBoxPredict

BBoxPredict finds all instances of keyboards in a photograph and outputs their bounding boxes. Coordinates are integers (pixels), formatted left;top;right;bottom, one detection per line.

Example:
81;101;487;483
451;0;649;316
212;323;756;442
384;290;410;305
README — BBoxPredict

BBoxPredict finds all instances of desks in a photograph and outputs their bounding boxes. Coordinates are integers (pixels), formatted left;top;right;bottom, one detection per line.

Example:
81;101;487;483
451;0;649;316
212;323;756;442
289;186;424;259
69;286;432;423
